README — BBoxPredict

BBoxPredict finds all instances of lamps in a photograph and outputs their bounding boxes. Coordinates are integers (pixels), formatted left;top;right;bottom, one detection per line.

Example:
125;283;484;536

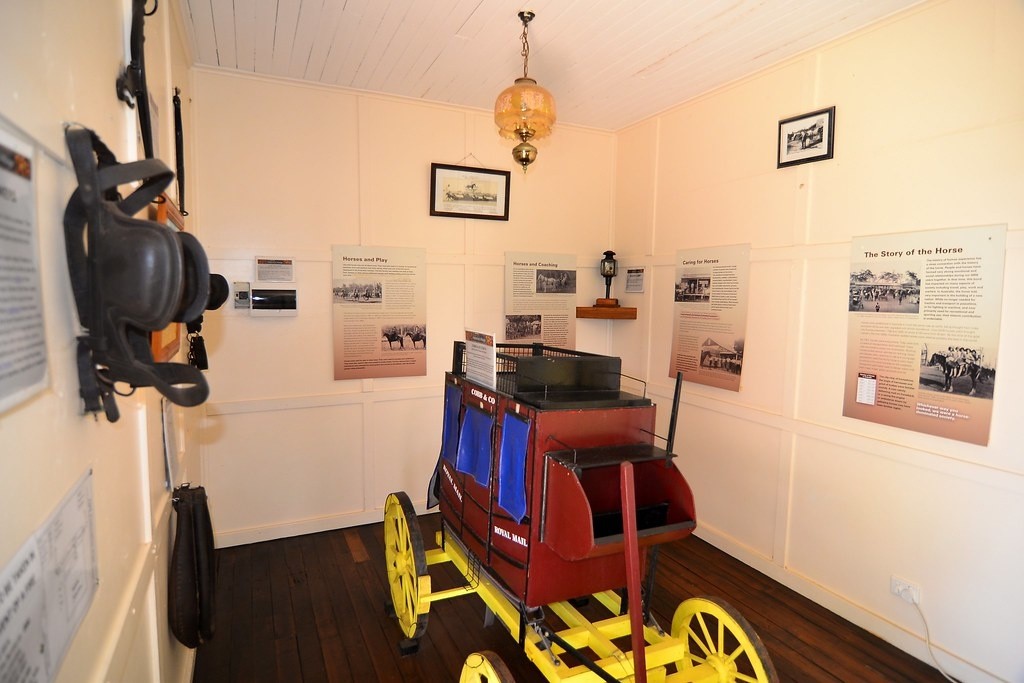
595;251;619;306
492;11;554;171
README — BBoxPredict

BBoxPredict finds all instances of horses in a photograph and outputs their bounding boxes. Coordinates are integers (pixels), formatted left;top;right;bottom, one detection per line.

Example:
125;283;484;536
538;274;560;293
382;333;404;351
404;332;426;349
335;293;382;302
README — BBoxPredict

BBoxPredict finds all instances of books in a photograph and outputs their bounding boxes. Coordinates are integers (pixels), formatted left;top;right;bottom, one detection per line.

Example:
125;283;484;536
465;329;496;389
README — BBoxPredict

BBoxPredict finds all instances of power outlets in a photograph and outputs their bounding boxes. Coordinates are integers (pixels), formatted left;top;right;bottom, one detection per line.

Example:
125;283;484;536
890;576;920;604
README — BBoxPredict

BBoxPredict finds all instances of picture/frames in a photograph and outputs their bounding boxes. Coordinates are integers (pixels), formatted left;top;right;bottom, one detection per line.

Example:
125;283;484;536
776;106;835;169
431;161;510;221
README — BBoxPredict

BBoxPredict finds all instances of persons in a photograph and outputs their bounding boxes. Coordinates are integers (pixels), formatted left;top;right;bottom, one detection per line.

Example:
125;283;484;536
390;326;397;335
709;355;742;374
850;287;914;305
415;325;420;335
685;282;694;294
507;321;537;339
559;273;567;287
798;131;807;149
875;303;881;312
944;346;978;377
